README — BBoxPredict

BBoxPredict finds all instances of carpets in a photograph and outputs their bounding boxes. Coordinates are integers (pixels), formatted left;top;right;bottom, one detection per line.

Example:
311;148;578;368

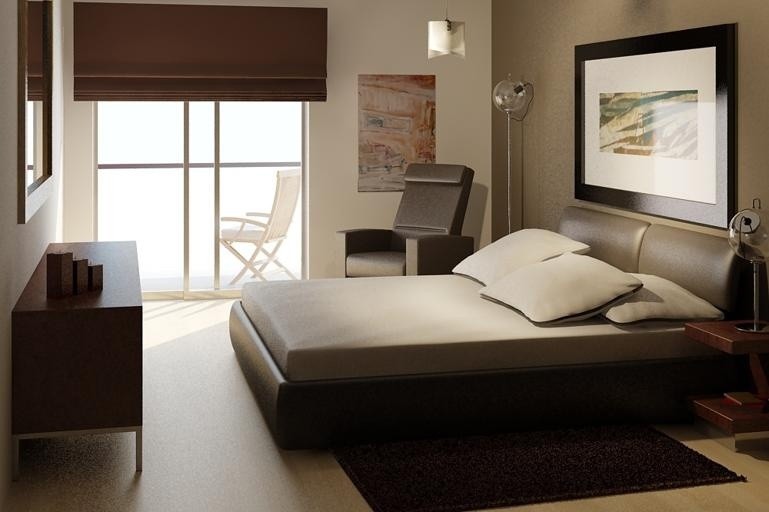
330;424;746;512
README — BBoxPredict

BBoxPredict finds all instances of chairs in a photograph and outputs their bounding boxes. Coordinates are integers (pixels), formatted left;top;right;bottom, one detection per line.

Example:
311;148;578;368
220;167;301;284
336;163;475;278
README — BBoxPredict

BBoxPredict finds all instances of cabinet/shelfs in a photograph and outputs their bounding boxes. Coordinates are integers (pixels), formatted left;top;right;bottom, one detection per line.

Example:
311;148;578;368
12;241;143;481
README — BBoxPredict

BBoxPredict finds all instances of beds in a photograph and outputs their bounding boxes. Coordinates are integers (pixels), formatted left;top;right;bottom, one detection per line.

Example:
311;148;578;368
230;205;742;449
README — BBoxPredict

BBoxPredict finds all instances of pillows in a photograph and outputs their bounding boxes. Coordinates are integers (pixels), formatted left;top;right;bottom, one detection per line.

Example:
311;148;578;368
600;273;724;326
480;251;643;325
452;228;591;287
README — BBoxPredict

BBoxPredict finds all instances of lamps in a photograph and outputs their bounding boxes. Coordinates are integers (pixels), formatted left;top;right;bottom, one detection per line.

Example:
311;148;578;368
428;0;465;60
493;74;534;234
727;198;769;333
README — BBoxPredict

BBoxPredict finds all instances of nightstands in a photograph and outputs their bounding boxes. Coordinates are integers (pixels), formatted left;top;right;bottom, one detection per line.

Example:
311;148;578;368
684;320;769;452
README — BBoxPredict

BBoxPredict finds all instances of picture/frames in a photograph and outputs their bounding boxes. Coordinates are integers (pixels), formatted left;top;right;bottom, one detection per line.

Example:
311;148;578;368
575;22;735;230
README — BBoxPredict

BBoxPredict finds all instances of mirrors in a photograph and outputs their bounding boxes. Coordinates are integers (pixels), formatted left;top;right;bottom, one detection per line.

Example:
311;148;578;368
17;0;54;224
